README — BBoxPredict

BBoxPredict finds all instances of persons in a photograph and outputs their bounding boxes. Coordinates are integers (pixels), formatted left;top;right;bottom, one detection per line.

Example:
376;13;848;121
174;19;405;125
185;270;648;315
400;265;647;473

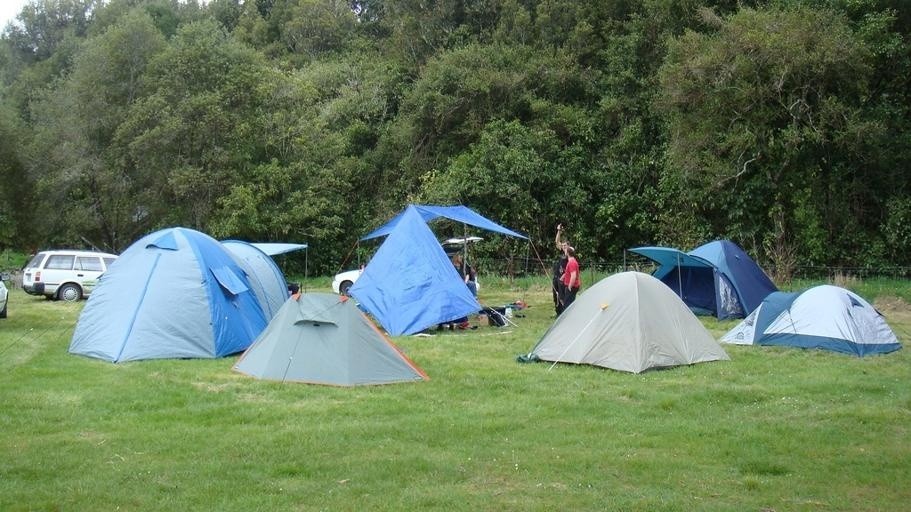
552;223;570;316
560;247;580;310
452;251;476;329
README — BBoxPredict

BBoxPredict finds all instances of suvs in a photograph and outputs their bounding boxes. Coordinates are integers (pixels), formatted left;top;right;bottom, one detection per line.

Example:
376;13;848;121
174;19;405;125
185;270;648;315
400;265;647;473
23;249;118;302
332;234;484;297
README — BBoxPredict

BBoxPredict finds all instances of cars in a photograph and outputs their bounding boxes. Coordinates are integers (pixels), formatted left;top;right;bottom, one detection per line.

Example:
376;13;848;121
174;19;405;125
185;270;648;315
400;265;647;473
1;274;8;321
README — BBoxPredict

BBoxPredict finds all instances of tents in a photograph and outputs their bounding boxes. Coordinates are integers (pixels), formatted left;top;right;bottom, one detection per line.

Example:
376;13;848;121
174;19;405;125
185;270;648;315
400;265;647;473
516;271;731;374
626;239;778;322
231;291;431;387
718;283;903;358
67;227;269;364
222;240;289;325
